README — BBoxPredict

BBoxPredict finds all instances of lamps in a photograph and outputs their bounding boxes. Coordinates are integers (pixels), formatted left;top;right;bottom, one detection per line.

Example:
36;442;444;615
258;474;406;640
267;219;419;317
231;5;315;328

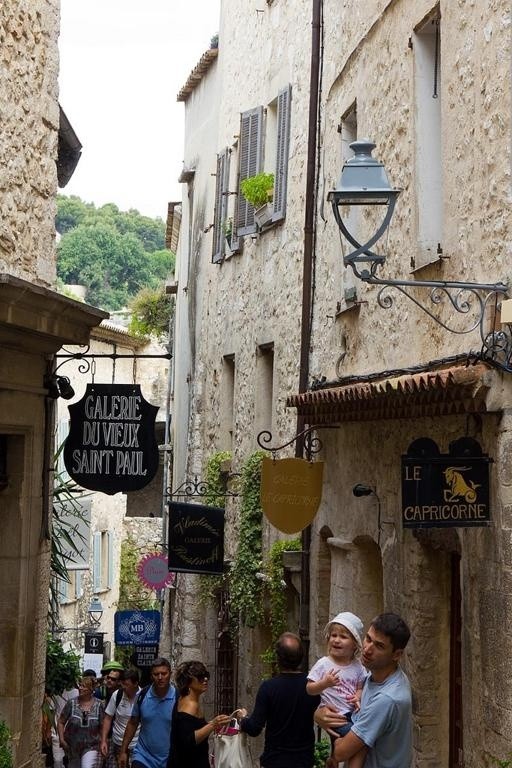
352;483;396;550
47;596;108;636
327;139;511;371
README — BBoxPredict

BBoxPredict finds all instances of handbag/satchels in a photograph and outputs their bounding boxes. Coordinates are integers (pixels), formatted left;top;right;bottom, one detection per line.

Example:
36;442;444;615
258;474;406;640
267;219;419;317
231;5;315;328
207;709;255;768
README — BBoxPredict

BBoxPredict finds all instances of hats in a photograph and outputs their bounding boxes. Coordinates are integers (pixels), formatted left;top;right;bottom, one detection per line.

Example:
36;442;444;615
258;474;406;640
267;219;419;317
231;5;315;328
323;610;364;651
101;661;124;673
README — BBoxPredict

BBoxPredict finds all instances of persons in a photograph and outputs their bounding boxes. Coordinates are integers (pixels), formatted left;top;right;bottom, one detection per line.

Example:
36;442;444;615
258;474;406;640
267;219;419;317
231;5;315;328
236;612;415;768
56;660;233;768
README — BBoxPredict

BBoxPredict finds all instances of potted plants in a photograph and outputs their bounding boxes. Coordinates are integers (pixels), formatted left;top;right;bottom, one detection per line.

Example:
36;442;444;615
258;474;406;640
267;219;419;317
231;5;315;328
221;216;233;249
240;171;274;228
